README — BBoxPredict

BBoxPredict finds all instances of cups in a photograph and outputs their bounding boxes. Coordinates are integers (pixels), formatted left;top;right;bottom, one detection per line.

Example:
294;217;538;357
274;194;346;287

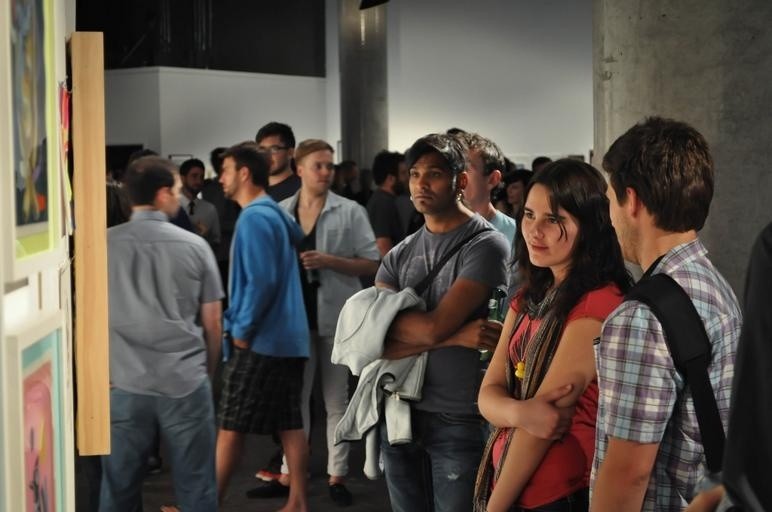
474;287;507;365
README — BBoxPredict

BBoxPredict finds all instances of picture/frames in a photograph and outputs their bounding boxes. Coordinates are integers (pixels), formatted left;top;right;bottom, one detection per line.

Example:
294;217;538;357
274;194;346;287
0;0;68;284
3;307;68;512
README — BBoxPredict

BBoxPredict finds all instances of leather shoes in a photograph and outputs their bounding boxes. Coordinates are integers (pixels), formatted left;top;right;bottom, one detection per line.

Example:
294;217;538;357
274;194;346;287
246;479;289;498
329;483;352;506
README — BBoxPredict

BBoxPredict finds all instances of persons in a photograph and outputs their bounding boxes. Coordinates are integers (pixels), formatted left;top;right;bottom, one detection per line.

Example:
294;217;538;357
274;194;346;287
685;224;770;511
472;158;637;511
78;122;554;511
588;114;740;511
